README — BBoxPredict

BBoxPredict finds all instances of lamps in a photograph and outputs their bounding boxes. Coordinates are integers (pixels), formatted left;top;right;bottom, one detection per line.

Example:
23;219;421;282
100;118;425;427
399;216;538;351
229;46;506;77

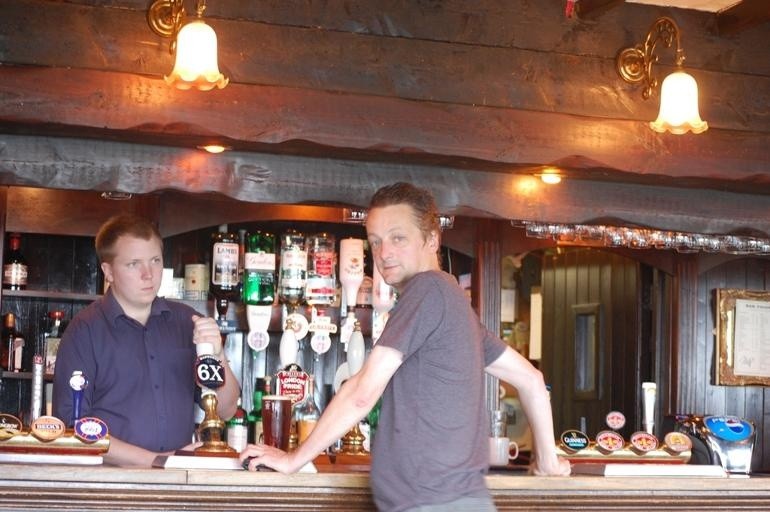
147;0;230;92
615;16;709;136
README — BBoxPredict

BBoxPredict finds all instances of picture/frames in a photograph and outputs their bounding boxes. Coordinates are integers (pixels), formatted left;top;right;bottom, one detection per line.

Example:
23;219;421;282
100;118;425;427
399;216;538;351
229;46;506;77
713;288;770;388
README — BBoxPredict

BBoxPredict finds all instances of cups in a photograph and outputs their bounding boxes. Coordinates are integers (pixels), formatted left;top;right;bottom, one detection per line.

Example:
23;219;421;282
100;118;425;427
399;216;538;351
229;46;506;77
506;219;770;255
488;435;519;467
261;395;290;452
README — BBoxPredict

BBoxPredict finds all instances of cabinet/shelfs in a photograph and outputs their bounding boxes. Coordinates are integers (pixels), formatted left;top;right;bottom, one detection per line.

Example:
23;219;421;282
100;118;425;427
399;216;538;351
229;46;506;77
0;184;502;466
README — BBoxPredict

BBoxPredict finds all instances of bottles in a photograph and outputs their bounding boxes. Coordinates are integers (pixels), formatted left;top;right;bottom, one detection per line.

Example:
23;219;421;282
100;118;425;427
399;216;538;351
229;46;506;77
298;378;320;446
226;377;273;453
4;233;64;376
211;228;364;320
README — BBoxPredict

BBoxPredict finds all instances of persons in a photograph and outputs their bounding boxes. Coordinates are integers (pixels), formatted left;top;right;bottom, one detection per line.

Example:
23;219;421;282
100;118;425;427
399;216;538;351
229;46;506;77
52;213;242;469
240;182;570;512
